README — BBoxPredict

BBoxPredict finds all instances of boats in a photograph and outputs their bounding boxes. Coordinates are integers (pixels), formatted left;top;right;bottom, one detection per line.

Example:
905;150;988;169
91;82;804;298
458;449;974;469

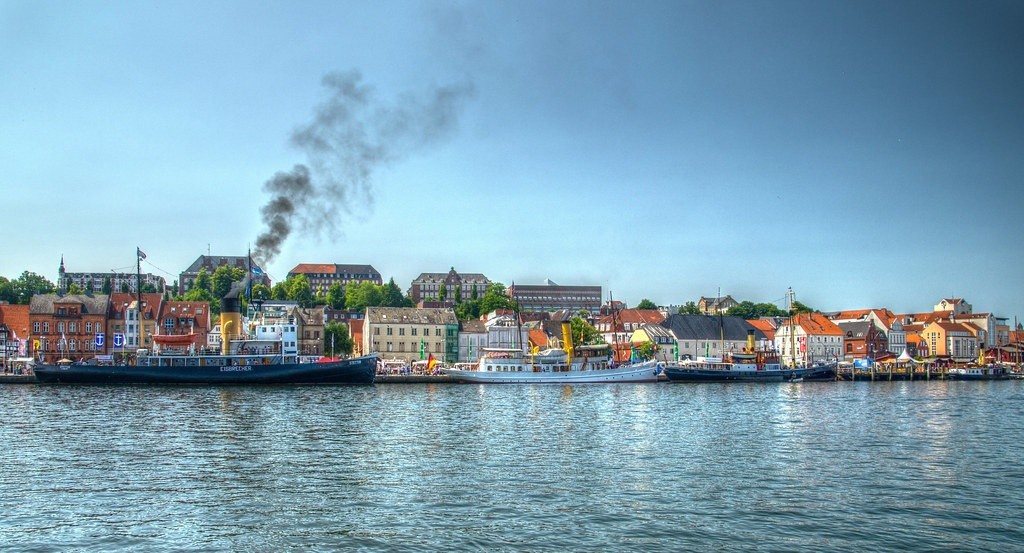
662;285;836;383
439;291;658;384
30;247;377;387
949;363;1011;379
